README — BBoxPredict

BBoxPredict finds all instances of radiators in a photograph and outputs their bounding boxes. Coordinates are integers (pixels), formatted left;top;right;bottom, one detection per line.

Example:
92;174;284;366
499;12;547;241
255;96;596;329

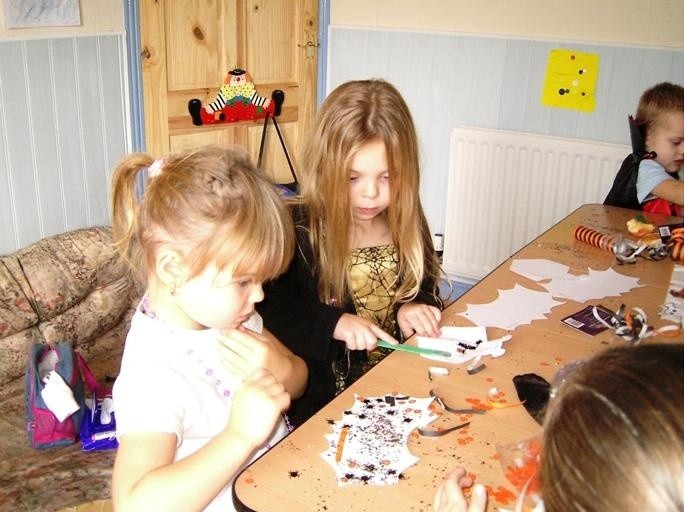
437;125;636;286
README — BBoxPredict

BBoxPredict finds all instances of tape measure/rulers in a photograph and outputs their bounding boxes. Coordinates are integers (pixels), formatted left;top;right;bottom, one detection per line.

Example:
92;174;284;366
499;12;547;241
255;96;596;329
377;340;452;358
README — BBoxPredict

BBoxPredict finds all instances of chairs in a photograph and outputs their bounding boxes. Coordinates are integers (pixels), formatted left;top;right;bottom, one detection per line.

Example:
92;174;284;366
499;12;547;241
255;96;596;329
0;224;148;512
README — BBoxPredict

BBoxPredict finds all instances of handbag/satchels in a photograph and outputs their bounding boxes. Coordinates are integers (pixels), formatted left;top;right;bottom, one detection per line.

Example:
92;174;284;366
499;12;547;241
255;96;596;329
24;342;82;449
275;183;305;225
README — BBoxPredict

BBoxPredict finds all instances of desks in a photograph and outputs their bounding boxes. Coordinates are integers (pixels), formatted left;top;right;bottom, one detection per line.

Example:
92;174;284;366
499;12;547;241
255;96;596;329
235;202;684;512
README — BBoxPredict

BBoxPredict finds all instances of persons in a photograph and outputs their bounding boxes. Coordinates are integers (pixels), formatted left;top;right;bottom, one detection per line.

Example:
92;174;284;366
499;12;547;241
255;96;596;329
250;78;454;430
430;343;683;512
104;140;309;512
603;81;684;218
